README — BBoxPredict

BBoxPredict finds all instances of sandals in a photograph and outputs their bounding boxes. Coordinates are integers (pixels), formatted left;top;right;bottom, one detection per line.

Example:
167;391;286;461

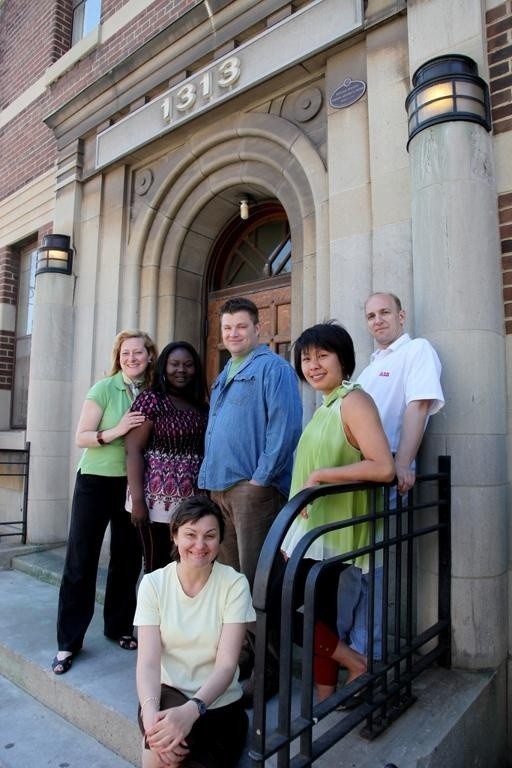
117;636;138;649
52;651;74;674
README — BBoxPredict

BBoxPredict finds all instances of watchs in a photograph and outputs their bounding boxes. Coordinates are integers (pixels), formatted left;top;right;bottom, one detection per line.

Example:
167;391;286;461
188;697;207;714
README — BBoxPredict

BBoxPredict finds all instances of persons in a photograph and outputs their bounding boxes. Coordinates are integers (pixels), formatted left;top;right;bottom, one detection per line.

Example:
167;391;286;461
131;496;249;768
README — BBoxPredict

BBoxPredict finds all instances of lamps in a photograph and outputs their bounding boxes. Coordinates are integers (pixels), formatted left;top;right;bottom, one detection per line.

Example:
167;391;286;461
405;52;493;152
33;233;73;275
